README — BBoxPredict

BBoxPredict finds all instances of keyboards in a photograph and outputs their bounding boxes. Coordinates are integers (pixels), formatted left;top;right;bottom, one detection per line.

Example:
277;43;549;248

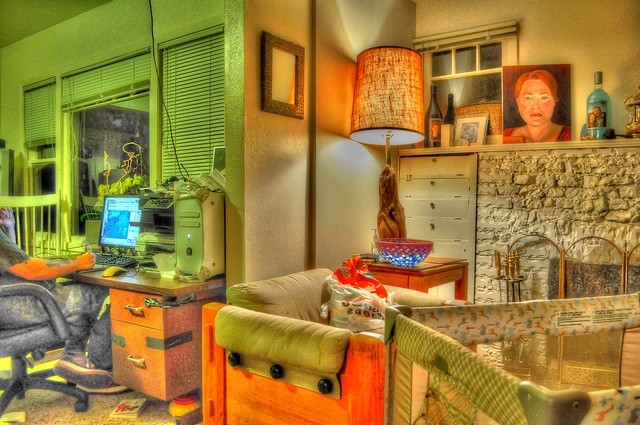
94;253;138;270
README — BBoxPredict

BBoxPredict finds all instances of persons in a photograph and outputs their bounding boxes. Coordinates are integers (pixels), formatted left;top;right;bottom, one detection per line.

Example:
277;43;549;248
431;122;441;140
588;112;596;127
593;105;607;127
503;70;571;142
0;205;127;393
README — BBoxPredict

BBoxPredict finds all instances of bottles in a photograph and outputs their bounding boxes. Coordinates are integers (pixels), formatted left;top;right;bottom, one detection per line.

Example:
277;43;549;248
441;93;456;146
425;84;443;147
586;70;611;139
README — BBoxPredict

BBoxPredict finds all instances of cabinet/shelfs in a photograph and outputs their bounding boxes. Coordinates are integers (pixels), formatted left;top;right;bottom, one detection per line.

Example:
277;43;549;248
394;149;476;301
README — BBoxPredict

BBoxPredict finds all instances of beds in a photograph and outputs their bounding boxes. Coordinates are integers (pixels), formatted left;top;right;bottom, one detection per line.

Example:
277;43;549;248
384;289;640;425
360;255;469;302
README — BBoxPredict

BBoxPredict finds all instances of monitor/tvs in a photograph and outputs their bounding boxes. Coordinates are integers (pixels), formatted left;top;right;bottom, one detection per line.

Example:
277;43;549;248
96;196;142;248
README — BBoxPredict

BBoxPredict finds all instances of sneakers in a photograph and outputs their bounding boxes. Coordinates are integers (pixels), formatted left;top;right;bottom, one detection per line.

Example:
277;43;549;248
53;353;113;389
75;381;128;393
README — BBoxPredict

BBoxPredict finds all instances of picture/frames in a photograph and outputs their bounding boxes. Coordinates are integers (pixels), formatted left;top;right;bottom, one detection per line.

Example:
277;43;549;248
260;27;305;118
451;115;489;146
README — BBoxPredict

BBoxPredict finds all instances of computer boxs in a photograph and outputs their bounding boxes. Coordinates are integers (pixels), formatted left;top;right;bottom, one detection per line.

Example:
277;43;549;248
174;190;224;283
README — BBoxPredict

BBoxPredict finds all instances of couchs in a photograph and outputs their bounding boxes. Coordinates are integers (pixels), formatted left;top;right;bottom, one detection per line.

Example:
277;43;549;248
203;270;442;424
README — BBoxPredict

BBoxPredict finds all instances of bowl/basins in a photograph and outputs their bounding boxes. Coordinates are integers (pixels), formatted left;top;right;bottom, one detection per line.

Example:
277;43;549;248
375;239;433;268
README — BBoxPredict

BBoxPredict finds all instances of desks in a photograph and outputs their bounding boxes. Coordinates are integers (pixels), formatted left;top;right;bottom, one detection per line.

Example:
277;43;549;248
32;256;225;400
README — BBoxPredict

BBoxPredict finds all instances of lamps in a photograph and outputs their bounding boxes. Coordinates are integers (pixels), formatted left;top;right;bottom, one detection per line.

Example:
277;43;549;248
348;46;427;242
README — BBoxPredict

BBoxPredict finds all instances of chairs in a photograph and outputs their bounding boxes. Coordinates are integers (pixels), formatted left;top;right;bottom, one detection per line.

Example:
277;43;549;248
0;283;91;415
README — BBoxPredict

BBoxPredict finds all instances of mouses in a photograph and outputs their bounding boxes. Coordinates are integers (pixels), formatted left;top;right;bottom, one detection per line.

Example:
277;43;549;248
101;265;127;278
